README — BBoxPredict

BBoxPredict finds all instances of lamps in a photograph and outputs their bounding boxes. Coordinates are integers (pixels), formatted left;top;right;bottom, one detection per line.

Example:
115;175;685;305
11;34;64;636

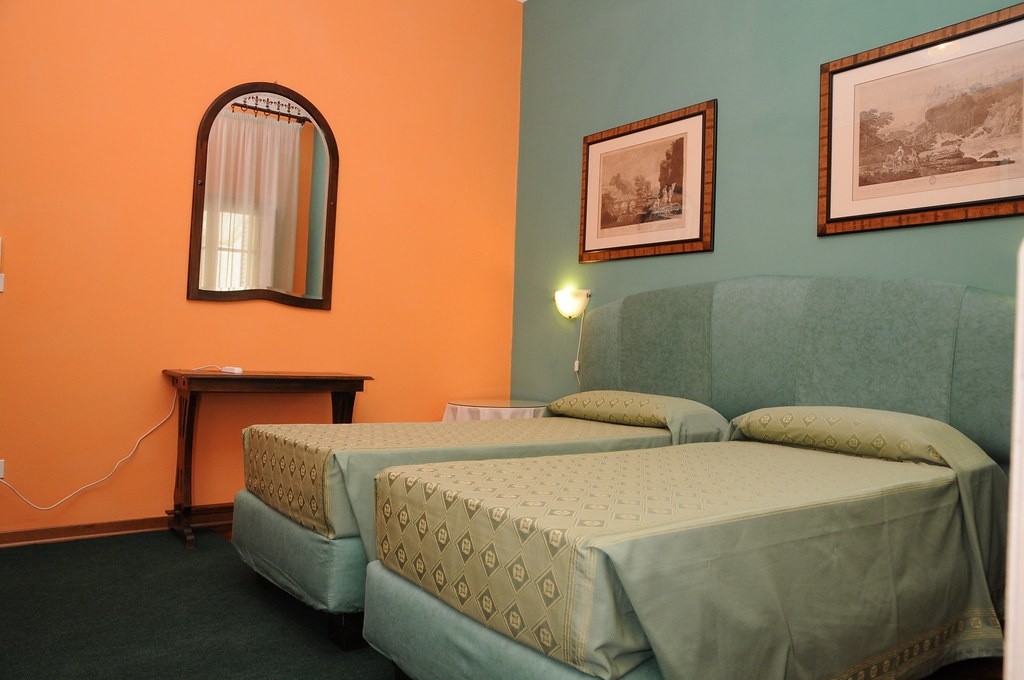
554;288;591;394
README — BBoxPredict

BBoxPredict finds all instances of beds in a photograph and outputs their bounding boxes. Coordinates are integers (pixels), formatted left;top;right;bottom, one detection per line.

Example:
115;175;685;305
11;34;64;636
250;274;1014;680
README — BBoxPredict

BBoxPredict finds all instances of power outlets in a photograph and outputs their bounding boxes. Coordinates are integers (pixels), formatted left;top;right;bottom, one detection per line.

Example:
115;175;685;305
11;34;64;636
0;460;5;479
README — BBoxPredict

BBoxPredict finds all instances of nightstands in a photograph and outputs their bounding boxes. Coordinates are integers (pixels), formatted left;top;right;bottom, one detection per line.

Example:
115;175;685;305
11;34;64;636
441;398;550;422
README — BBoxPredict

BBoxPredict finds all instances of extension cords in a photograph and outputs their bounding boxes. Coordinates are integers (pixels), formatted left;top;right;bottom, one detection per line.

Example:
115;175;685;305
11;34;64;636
222;367;242;373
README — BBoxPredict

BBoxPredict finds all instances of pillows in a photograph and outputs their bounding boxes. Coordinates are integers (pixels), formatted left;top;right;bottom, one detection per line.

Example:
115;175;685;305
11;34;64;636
725;405;974;468
544;387;716;429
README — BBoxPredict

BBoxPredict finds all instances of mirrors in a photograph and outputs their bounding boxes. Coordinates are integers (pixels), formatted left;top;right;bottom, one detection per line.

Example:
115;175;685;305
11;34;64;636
183;81;339;311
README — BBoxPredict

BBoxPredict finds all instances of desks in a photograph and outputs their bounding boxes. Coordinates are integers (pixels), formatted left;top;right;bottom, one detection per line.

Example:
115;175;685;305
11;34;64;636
161;368;375;550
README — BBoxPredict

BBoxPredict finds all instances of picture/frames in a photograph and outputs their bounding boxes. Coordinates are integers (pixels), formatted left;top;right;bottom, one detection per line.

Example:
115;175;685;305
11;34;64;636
573;98;714;264
816;3;1024;236
